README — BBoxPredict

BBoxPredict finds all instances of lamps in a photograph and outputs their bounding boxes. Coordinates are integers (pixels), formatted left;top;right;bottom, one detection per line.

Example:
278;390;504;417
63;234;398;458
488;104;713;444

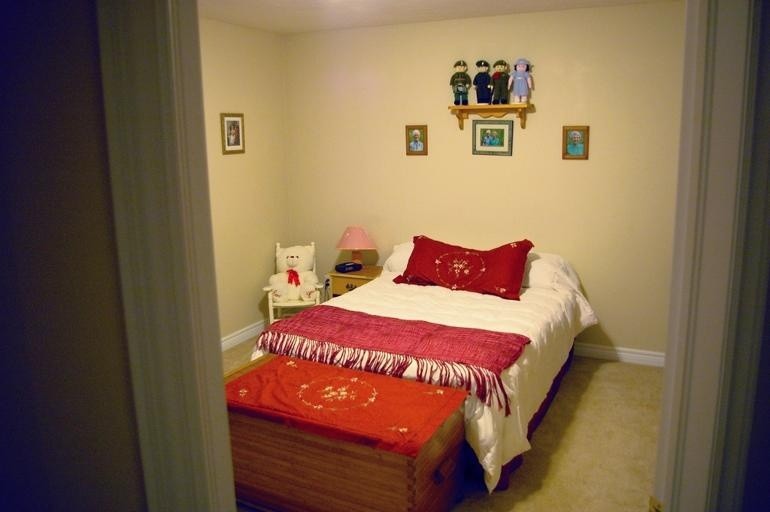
338;227;375;269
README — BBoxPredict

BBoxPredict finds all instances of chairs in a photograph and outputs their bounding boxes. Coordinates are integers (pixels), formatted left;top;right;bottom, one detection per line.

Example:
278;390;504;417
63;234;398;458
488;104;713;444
266;242;321;329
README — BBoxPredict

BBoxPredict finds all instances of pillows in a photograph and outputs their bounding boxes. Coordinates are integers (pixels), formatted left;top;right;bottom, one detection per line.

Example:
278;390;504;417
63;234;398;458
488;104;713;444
392;236;534;302
383;242;414;272
521;252;582;290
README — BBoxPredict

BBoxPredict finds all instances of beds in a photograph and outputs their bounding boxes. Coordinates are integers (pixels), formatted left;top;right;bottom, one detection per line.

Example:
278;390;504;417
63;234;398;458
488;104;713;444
253;236;597;495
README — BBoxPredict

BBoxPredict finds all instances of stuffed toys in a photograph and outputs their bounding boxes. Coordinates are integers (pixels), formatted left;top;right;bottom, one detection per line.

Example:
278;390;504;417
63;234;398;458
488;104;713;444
268;245;320;303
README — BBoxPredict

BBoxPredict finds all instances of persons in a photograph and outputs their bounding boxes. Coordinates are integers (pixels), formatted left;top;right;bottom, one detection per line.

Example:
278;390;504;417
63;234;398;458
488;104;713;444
228;120;240;146
566;130;584;156
410;129;423;151
449;60;473;106
472;60;492;104
491;130;501;147
482;129;492;146
508;58;532;104
490;59;510;105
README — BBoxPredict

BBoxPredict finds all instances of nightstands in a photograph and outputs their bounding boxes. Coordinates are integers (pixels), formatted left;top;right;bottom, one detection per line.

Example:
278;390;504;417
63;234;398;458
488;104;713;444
327;265;381;298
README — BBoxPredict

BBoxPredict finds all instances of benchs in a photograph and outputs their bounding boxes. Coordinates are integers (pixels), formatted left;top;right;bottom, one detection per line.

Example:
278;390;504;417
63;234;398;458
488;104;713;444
224;352;470;510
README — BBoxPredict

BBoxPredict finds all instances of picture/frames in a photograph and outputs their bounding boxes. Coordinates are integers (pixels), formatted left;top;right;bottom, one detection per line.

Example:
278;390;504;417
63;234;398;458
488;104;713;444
472;119;513;157
406;125;428;156
561;125;590;160
221;112;245;156
